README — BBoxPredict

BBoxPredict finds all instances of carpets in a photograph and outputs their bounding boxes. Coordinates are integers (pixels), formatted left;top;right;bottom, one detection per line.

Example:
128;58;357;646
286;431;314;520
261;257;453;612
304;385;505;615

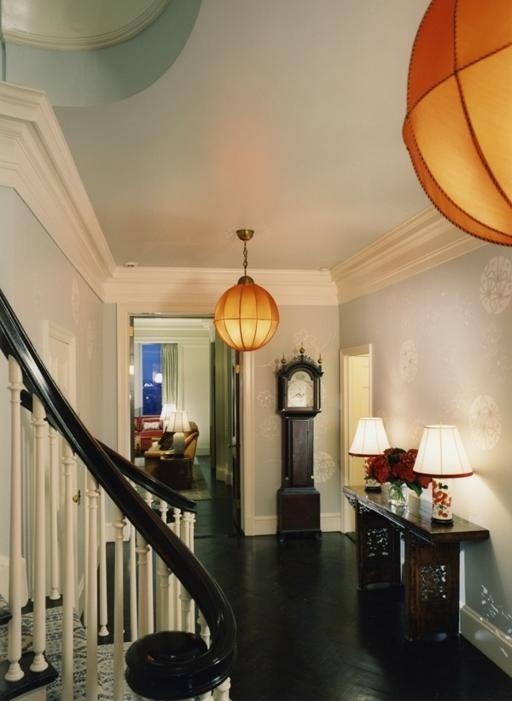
135;455;213;501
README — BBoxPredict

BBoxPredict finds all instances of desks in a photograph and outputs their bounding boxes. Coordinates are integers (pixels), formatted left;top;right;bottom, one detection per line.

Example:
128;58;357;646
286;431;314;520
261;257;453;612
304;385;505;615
343;486;489;643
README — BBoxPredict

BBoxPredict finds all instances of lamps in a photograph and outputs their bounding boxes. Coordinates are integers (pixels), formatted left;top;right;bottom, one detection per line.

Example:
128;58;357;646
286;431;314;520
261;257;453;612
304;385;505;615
166;411;191;455
401;0;511;250
212;228;278;352
412;425;473;525
348;416;390;491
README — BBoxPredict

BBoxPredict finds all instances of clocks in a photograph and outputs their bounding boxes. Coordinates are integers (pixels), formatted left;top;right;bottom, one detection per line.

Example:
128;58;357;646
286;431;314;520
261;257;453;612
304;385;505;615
278;340;324;416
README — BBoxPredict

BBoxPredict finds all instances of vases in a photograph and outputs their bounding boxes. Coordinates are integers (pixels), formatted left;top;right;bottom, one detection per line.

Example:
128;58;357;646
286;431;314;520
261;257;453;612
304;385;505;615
387;484;407;507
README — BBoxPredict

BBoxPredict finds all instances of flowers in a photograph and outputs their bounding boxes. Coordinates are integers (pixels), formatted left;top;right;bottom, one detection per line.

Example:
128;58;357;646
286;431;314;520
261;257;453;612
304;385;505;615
363;447;432;501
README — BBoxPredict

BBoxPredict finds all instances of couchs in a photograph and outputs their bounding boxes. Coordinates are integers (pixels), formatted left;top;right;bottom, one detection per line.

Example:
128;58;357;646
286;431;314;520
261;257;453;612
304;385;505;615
134;417;163;450
144;421;199;476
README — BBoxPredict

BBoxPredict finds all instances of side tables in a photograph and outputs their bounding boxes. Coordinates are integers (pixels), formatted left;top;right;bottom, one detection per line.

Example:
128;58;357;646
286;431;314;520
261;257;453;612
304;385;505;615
159;455;191;489
